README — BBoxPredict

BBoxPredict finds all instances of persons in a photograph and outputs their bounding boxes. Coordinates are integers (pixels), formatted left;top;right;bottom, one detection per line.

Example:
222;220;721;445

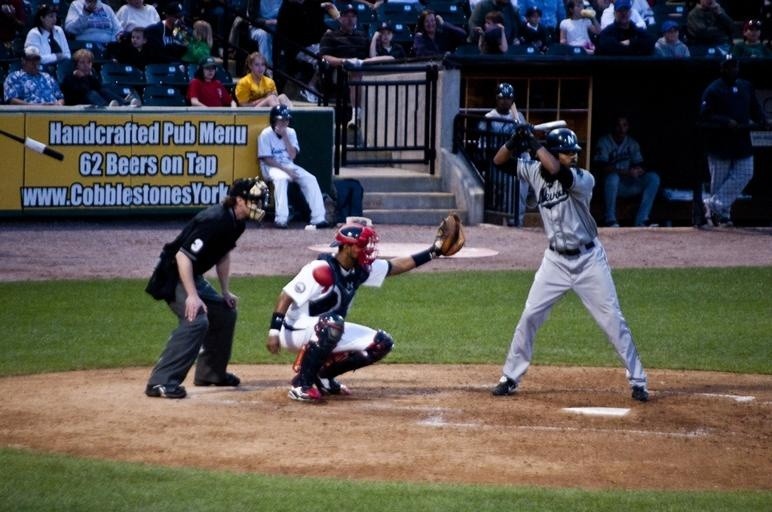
1;1;769;230
265;213;463;407
146;175;273;398
488;118;651;401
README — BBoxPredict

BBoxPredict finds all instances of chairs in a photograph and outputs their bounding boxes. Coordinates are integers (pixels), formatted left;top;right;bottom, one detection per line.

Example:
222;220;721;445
57;58;235;108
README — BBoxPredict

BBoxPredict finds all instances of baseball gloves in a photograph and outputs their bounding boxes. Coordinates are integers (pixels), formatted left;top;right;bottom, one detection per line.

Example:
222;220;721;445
433;215;465;256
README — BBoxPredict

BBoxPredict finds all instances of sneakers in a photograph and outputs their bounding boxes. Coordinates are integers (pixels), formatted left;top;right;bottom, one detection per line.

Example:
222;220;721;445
632;385;649;401
275;222;288;229
289;385;323;403
700;219;711;226
642;223;658;228
195;373;240;386
146;383;187;399
719;220;733;228
608;222;618;228
316;222;331;228
492;376;516;397
321;379;349;395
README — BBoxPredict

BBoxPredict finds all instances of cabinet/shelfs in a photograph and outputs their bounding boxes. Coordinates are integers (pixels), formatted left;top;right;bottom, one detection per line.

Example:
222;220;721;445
460;75;592;177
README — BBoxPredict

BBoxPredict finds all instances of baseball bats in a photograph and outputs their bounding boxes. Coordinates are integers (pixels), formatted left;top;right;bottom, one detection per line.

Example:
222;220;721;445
511;102;519;124
533;120;568;131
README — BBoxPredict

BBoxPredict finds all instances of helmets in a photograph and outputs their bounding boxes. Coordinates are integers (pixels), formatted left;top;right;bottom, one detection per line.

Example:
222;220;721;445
227;175;269;222
547;128;581;152
330;222;379;266
495;84;512;99
270;104;292;121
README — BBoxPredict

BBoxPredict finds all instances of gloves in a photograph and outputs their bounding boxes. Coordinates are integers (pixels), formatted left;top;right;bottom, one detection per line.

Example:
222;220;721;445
506;123;542;154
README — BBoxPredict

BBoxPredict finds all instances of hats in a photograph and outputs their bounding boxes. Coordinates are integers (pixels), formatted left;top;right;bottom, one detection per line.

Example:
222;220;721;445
745;20;762;32
202;57;215;68
663;21;677;32
22;46;41;60
722;54;740;65
614;0;632;10
378;21;396;32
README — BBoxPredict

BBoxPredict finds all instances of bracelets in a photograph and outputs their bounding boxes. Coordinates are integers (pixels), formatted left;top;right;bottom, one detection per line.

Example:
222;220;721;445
410;249;433;267
270;310;283;328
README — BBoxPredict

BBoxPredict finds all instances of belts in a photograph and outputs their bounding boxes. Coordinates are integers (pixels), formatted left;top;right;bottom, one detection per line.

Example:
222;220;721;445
548;242;594;255
